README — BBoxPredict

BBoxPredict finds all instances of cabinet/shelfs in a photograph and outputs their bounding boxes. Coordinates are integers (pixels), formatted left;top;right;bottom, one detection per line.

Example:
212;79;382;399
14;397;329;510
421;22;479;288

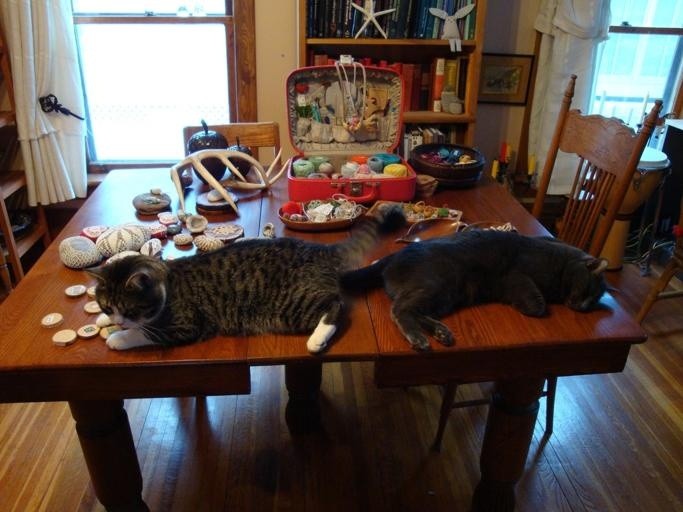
297;0;487;161
1;172;53;285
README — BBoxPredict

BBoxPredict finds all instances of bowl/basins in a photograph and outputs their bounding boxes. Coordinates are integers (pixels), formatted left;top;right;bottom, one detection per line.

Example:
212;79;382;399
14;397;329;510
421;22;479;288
410;143;486;188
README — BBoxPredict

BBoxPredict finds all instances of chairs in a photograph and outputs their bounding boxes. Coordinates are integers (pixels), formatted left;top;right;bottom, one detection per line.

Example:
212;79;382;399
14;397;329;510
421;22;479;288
430;74;662;455
183;123;281;416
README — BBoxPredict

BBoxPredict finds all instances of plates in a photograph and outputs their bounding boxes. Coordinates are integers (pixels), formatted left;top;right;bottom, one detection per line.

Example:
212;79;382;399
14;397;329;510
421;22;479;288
396;218;460;245
365;199;463;227
278;199;362;232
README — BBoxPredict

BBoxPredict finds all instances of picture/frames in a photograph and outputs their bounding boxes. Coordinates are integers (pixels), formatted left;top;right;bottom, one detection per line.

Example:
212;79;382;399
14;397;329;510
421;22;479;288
478;52;535;107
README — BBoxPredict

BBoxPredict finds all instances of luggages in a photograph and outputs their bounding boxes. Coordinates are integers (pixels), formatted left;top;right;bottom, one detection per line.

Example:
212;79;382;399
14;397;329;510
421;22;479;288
283;62;420;207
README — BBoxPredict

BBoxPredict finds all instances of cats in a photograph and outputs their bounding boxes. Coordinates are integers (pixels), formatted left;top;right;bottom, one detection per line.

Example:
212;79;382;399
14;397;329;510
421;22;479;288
338;229;612;353
82;202;408;355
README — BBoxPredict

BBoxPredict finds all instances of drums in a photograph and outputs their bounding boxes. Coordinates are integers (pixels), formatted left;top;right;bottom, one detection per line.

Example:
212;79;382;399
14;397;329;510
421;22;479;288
585;145;671;270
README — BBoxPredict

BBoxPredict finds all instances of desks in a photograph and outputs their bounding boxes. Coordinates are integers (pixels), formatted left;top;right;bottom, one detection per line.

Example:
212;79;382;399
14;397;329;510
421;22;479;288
1;167;648;512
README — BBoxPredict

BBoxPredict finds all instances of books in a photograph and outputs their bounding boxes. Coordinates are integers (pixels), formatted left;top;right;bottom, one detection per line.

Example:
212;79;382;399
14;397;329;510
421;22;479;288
305;0;477;165
